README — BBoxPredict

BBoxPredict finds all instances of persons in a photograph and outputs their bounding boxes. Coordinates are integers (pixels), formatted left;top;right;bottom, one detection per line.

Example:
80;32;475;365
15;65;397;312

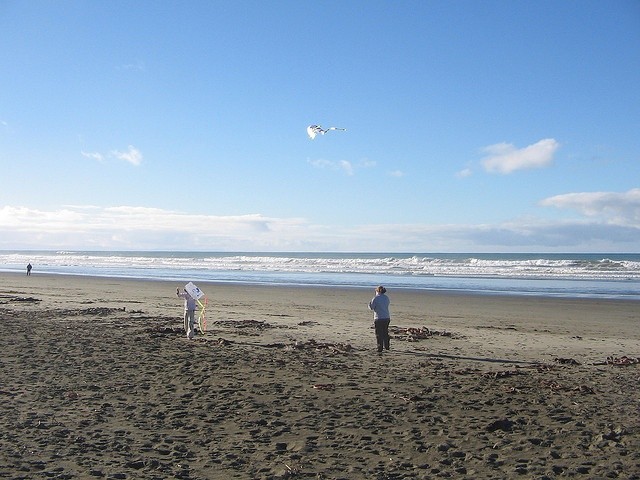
175;287;196;340
27;263;32;276
368;286;391;353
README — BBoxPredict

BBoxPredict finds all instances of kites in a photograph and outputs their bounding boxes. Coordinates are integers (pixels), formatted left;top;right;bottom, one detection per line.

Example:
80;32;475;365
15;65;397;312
185;281;208;335
307;124;349;139
